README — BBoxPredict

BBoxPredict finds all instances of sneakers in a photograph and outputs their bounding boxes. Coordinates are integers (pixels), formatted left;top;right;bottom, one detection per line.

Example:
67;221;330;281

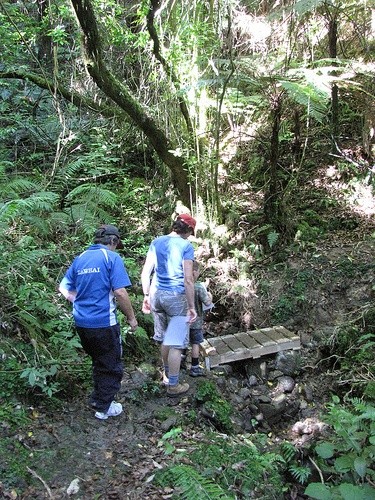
163;375;169;385
189;367;205;377
168;380;190;394
89;399;123;419
180;360;187;370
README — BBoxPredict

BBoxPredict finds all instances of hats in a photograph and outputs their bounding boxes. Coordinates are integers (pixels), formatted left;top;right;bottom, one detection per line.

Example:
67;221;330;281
178;214;196;236
96;226;121;239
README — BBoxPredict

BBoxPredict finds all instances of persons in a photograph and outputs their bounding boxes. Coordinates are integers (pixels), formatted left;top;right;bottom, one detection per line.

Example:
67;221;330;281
180;260;213;377
141;213;198;395
59;225;138;420
